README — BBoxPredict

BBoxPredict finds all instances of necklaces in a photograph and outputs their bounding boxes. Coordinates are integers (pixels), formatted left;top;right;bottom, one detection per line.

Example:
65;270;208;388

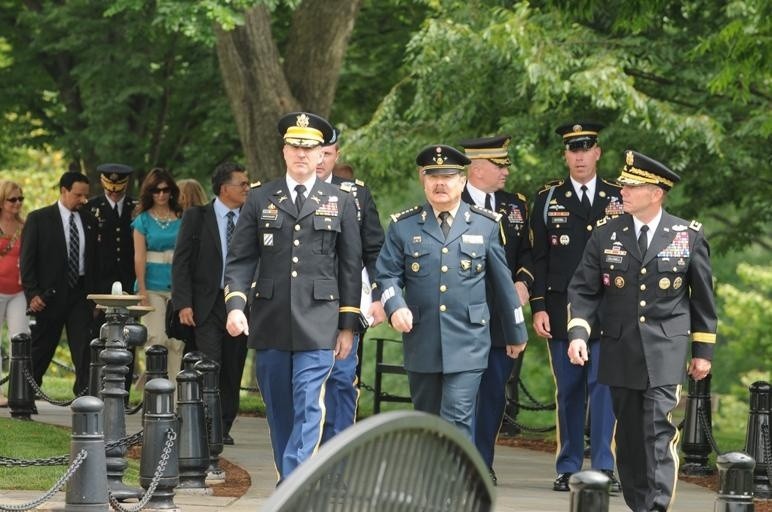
153;212;173;229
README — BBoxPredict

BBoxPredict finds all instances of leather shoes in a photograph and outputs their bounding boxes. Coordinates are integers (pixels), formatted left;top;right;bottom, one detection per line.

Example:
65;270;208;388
598;468;623;497
552;472;574;492
222;432;235;445
487;465;499;487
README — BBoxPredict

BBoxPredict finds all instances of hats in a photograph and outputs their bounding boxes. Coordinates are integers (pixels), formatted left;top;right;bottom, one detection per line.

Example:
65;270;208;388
319;125;340;147
615;149;681;193
96;163;134;193
276;110;334;149
415;144;471;176
552;122;605;151
460;134;514;167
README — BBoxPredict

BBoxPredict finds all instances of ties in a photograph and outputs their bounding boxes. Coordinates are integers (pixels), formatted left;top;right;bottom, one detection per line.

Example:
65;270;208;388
485;193;493;211
293;184;306;215
580;184;592;211
637;225;650;263
438;211;450;240
66;213;79;289
225;211;236;255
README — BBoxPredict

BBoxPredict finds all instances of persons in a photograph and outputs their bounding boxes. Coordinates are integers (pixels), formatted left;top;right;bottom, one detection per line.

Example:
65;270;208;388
0;178;28;370
174;178;206;208
528;121;625;494
130;167;184;412
333;165;354;179
82;163;142;414
316;126;386;448
172;159;251;446
458;135;533;485
20;173;99;416
565;149;715;512
224;110;363;487
372;143;529;447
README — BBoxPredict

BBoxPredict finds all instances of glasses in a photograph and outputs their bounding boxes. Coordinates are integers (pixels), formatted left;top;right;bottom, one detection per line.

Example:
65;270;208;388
151;187;171;194
6;196;25;203
